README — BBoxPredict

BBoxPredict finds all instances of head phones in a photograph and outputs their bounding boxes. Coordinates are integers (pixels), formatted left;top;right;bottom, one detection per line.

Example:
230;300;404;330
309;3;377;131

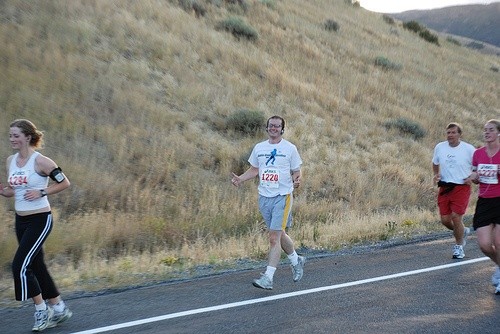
265;118;285;135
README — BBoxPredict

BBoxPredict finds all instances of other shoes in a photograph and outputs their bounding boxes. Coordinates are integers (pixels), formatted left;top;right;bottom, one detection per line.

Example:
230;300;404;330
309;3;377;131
492;268;500;294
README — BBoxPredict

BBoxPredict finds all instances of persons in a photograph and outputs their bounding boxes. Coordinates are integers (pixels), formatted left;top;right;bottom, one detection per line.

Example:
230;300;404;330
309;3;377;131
431;122;477;260
470;119;500;295
0;118;74;331
231;115;306;289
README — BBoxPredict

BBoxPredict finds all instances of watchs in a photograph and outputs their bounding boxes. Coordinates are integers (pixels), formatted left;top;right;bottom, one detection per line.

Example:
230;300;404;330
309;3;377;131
39;188;46;197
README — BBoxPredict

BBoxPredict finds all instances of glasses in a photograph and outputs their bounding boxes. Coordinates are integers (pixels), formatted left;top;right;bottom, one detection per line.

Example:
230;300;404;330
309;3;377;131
268;124;281;128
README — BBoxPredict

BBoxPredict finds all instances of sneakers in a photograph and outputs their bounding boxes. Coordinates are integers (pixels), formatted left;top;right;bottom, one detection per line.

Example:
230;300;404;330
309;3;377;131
32;306;54;331
453;245;465;259
47;307;73;328
252;273;273;289
463;227;470;247
291;256;306;281
